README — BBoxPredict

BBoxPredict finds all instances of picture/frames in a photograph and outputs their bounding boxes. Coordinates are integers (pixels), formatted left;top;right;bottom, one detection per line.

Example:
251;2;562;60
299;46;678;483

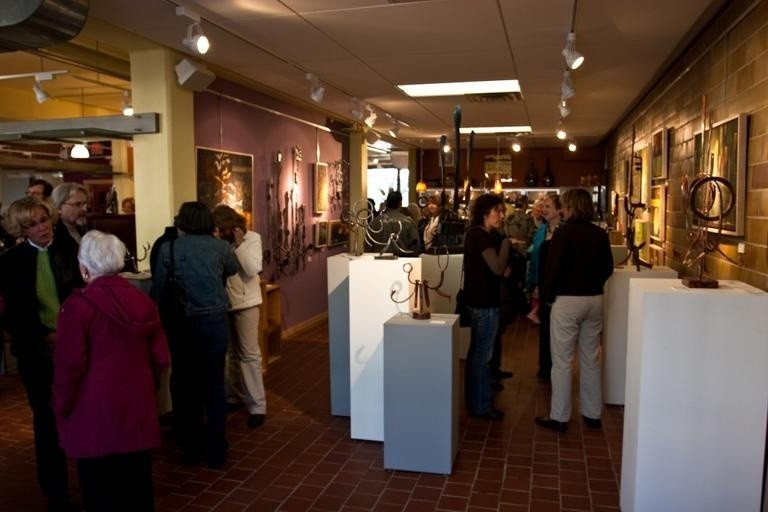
691;111;748;238
313;162;350;247
196;145;254;232
607;126;669;269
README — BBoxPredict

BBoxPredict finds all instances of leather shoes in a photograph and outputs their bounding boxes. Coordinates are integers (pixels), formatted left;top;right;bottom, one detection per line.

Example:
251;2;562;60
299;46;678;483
247;414;263;427
480;371;513;420
581;416;602;428
534;416;568;432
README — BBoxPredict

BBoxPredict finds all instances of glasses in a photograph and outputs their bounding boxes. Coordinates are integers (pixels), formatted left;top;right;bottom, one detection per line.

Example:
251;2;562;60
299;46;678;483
63;201;87;208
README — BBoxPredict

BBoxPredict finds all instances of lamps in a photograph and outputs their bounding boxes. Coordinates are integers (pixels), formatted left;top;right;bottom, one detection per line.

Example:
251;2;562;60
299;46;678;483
32;74;52;103
411;134;506;198
181;21;211;56
120;95;135;117
555;33;585;140
434;133;580;153
69;85;94;159
303;69;412;138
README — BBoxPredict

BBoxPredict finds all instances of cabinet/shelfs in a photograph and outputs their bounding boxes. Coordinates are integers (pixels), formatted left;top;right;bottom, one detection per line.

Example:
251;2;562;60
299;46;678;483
256;279;285;376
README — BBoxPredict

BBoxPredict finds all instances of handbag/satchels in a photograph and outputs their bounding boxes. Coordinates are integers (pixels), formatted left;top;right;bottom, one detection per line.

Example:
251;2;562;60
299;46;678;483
150;238;181;326
